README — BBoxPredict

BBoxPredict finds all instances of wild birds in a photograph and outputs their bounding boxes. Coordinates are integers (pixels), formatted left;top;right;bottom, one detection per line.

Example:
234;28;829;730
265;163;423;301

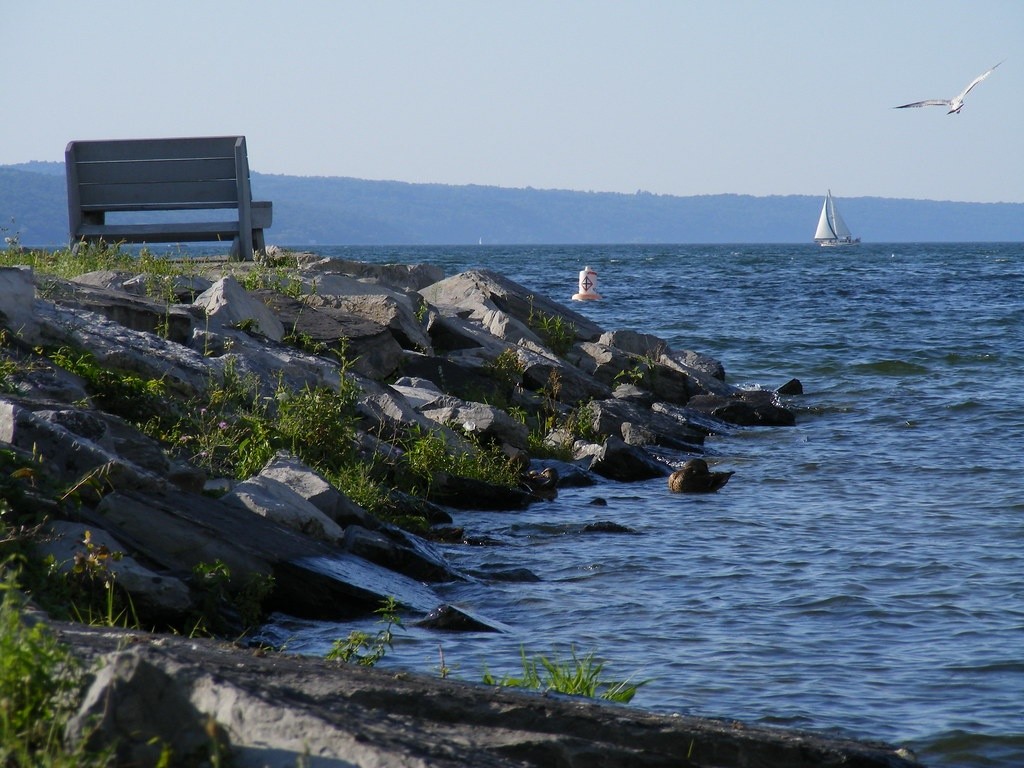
889;58;1007;114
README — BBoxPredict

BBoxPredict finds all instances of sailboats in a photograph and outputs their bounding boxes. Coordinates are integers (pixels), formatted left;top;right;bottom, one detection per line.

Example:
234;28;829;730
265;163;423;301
814;188;861;247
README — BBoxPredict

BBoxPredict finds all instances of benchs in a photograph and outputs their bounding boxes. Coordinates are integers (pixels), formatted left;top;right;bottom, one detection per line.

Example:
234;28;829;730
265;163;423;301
65;136;272;266
